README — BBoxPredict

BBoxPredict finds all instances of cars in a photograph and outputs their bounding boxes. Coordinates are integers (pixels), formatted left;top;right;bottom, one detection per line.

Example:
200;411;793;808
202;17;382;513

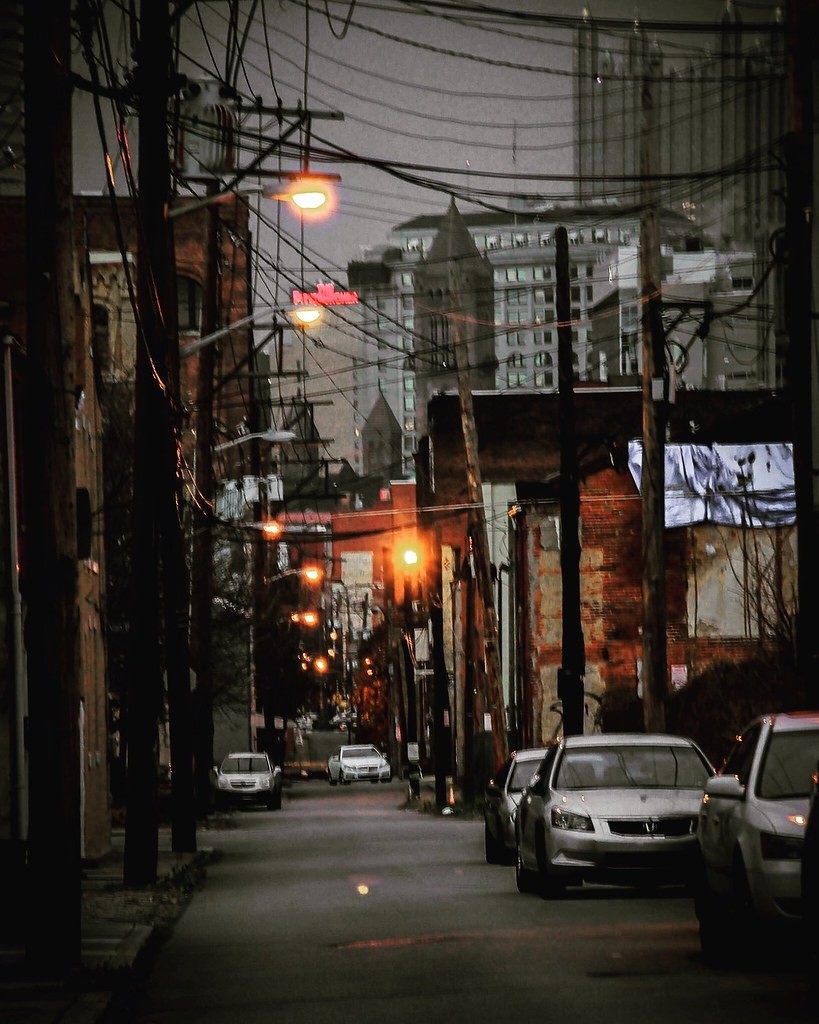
481;746;608;867
324;743;392;787
689;711;819;967
513;731;717;900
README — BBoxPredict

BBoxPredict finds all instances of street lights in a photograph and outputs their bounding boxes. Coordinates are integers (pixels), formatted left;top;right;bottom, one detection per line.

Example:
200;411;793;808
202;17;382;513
168;170;343;226
175;300;329;362
211;429;297;460
215;520;330;678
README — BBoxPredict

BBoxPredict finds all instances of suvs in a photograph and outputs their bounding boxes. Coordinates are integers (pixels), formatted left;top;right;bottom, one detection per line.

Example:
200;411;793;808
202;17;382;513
213;750;283;811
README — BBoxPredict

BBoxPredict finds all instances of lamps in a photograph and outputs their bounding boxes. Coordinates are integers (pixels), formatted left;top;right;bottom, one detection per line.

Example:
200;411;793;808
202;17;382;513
169;179;330;583
